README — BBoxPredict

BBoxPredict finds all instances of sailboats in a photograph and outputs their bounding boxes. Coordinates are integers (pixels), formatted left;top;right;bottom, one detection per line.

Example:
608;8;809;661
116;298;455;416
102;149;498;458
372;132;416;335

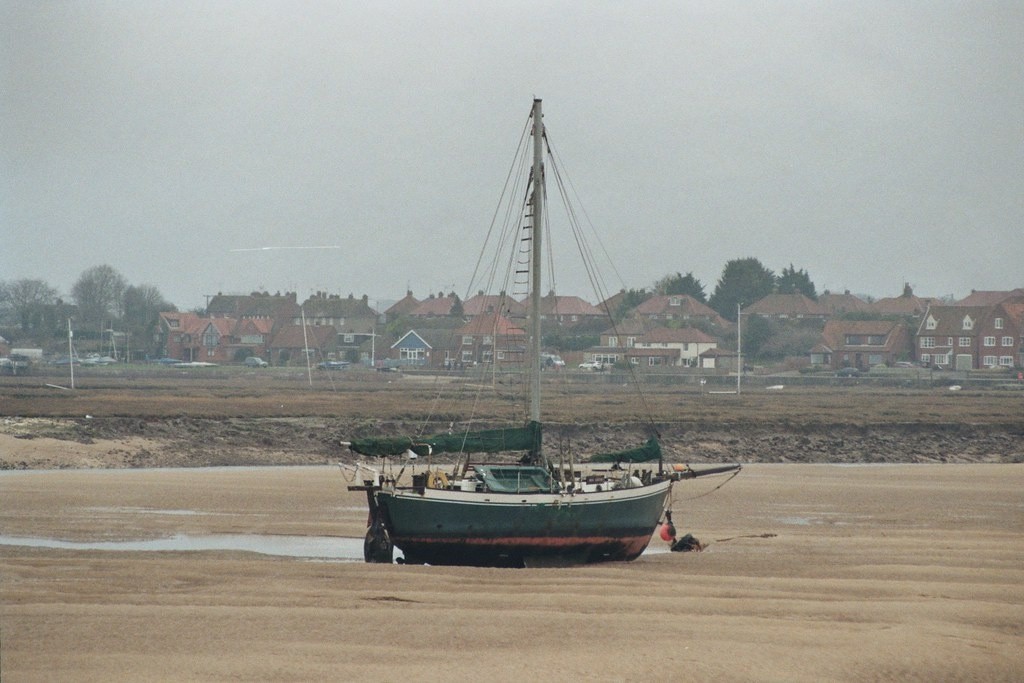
339;96;742;568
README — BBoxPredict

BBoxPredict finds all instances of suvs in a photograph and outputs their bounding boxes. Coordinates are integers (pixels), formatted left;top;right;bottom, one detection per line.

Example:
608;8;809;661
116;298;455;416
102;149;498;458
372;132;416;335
834;367;860;377
578;361;603;371
245;357;268;369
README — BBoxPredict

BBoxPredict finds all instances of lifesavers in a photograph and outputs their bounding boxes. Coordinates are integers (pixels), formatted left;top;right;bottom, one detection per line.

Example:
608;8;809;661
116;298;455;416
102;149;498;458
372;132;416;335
428;472;449;489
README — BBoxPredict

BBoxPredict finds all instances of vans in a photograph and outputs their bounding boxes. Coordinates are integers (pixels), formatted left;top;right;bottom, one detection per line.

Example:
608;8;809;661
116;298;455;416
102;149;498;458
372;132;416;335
539;354;565;368
